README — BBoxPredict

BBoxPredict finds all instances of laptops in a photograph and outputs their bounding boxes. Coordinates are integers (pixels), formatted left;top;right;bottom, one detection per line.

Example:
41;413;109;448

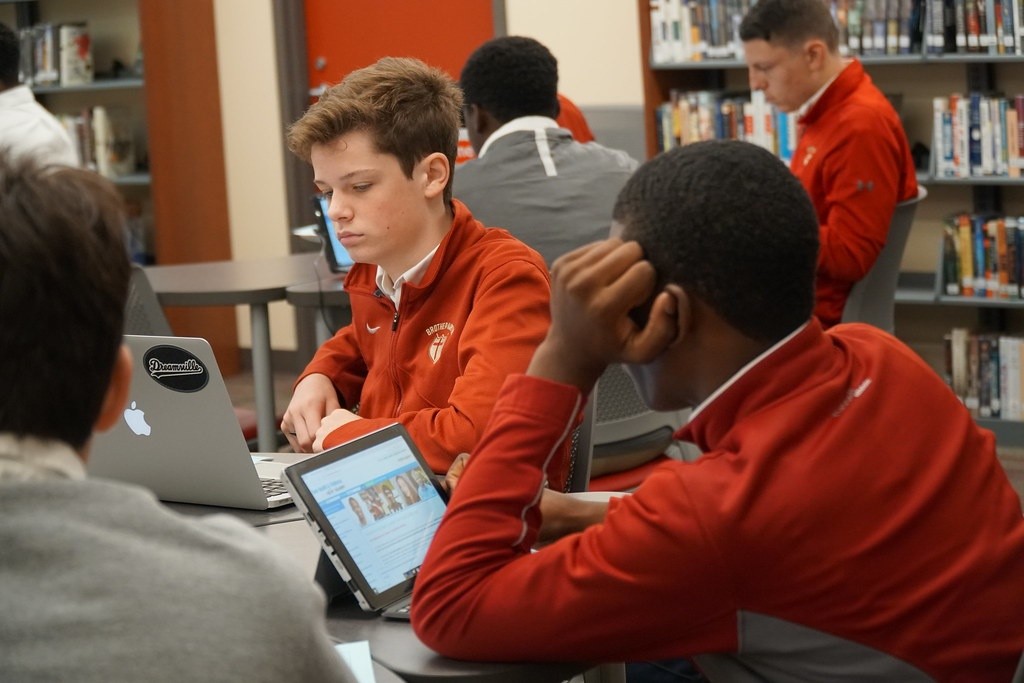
279;421;451;622
313;193;355;273
88;334;295;511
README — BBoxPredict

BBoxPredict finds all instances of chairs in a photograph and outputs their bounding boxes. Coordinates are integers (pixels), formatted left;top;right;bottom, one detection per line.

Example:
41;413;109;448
567;362;703;494
840;184;927;337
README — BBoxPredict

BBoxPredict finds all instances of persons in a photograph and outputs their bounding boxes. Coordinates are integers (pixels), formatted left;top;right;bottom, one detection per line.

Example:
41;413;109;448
0;140;356;683
0;26;82;181
740;0;919;332
281;56;590;494
452;35;693;479
410;138;1023;683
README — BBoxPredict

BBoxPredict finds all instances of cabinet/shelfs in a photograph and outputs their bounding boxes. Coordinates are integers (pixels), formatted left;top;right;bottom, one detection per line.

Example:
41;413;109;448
0;0;151;267
635;0;1024;451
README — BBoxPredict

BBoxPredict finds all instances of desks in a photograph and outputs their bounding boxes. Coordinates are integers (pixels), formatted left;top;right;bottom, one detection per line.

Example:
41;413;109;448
231;518;624;682
138;253;351;455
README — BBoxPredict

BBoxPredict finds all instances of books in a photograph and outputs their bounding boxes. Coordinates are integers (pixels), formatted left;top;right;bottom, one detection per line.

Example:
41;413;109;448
646;0;1024;423
49;105;135;178
8;21;94;89
348;469;437;525
